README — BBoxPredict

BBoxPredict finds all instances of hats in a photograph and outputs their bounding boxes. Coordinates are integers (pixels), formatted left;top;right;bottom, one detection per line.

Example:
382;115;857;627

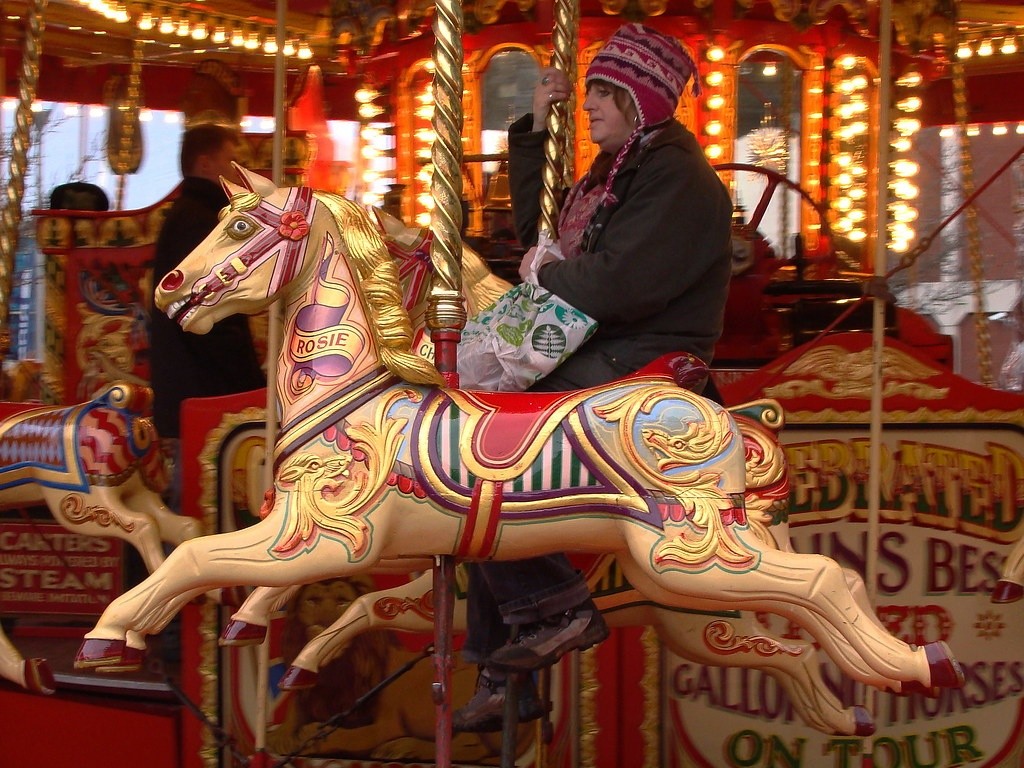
585;21;700;201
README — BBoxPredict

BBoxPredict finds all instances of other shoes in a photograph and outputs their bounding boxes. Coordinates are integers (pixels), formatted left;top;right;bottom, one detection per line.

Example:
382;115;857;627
133;639;183;681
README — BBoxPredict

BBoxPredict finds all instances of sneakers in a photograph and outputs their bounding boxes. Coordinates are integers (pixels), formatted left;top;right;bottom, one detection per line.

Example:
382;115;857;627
453;669;545;729
485;596;609;673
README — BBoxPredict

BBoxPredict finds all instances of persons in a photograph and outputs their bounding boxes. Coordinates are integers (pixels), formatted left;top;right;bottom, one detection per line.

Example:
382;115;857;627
142;126;267;437
452;22;735;736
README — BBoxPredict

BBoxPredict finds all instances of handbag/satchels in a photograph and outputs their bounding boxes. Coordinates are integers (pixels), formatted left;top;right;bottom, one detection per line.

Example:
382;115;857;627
455;261;598;391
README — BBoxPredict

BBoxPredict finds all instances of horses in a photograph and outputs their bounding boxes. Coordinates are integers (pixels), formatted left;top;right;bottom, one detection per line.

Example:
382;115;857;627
0;152;970;746
